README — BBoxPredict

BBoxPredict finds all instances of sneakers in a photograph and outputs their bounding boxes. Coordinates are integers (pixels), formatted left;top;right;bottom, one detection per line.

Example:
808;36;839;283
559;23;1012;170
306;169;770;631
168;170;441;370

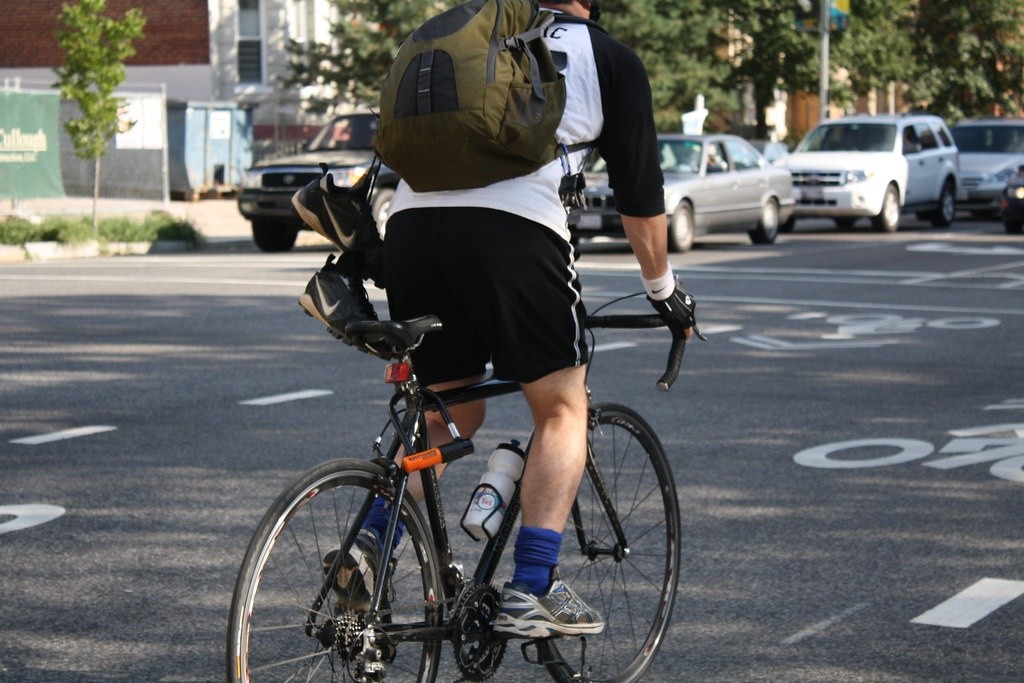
323;528;395;664
491;564;605;638
292;162;393;361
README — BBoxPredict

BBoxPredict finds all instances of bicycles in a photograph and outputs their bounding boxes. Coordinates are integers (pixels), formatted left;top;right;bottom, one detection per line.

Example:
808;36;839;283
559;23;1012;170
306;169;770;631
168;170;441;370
220;294;710;683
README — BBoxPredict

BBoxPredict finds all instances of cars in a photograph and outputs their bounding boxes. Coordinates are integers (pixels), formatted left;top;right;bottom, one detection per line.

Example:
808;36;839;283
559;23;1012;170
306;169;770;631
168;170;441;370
239;108;383;253
564;133;801;255
1000;165;1023;233
945;120;1023;219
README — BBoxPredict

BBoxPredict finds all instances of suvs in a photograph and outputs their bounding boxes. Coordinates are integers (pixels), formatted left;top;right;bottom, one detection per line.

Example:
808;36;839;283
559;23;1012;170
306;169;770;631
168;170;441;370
776;113;961;230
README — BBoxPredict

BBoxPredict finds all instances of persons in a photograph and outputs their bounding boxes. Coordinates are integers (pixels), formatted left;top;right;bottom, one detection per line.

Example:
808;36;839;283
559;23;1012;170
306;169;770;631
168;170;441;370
323;0;697;659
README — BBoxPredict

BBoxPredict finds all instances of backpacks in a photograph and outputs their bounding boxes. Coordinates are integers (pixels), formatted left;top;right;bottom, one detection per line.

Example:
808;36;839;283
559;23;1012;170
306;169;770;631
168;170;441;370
370;0;566;192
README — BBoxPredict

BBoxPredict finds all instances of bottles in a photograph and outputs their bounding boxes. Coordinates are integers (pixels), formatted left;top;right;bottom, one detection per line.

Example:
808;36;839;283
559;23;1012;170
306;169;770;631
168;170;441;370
461;436;526;539
